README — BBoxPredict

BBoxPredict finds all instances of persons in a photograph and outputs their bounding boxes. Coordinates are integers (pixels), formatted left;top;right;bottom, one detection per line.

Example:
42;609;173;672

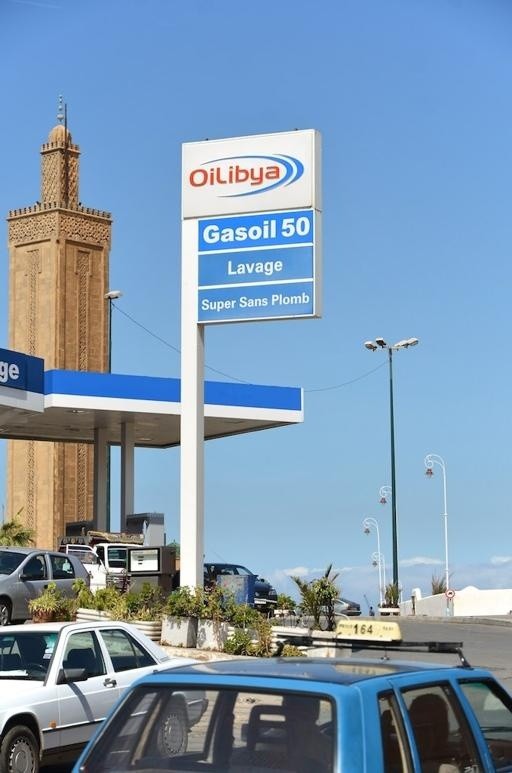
255;695;332;766
408;694;459;773
369;607;375;616
81;552;96;564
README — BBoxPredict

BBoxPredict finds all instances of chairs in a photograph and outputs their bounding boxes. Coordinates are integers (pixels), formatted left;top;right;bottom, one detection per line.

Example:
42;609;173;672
230;704;331;771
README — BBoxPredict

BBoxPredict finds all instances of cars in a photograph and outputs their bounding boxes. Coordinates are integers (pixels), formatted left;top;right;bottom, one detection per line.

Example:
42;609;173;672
0;545;91;626
318;597;361;616
0;621;210;773
172;561;277;613
75;655;512;773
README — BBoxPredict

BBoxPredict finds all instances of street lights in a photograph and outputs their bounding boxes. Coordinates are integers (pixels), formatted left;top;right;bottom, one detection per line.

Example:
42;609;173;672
364;452;451;598
363;332;422;603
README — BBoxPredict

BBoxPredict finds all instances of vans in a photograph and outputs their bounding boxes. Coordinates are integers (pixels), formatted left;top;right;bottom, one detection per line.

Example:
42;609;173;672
66;541;144;595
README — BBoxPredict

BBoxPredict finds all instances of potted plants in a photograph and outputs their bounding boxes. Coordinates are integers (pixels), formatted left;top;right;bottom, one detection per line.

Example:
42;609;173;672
25;577;277;659
